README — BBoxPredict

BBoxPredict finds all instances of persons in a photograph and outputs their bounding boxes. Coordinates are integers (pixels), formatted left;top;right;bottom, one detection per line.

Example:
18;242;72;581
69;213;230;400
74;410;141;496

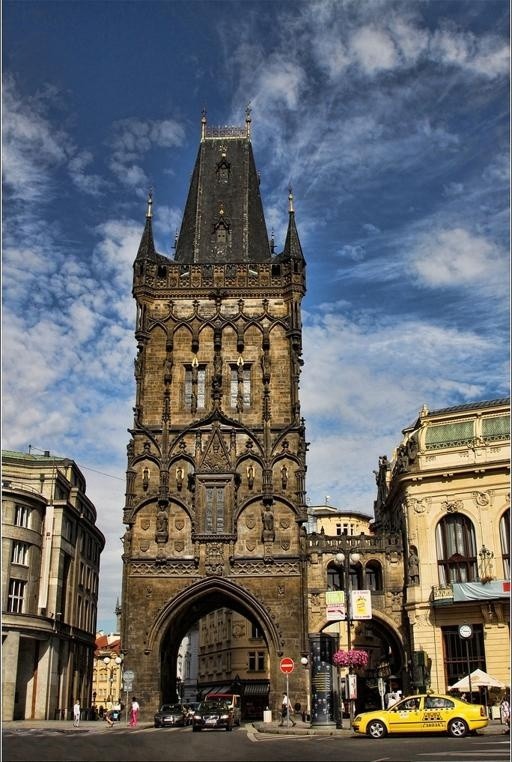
102;697;114;727
276;691;297;726
387;694;397;708
73;699;80;727
460;691;466;700
127;695;140;726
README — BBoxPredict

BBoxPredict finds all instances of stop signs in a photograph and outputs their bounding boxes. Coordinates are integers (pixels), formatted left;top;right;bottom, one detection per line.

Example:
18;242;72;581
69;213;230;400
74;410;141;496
279;657;294;674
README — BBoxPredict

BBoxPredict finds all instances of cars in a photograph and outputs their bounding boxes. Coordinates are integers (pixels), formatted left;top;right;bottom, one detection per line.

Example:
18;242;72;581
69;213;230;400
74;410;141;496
107;710;121;721
155;694;242;732
351;695;491;740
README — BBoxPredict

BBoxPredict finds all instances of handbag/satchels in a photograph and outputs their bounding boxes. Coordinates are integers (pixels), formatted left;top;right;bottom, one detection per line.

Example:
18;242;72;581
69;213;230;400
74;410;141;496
282;710;287;718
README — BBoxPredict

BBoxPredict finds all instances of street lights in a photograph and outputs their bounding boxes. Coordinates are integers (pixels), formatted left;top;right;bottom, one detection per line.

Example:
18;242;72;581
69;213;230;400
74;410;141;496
103;655;123;704
92;692;98;706
332;544;362;731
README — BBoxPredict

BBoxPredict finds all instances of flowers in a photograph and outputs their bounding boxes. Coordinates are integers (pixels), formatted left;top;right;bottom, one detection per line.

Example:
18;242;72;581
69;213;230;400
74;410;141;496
332;649;369;668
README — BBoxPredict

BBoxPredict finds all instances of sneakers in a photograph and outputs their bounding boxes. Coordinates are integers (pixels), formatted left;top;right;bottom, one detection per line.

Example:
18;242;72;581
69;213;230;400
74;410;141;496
278;722;296;726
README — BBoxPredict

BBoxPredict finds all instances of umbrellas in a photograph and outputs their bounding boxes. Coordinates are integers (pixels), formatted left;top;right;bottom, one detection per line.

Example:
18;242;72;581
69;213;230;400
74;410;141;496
450;667;504;703
